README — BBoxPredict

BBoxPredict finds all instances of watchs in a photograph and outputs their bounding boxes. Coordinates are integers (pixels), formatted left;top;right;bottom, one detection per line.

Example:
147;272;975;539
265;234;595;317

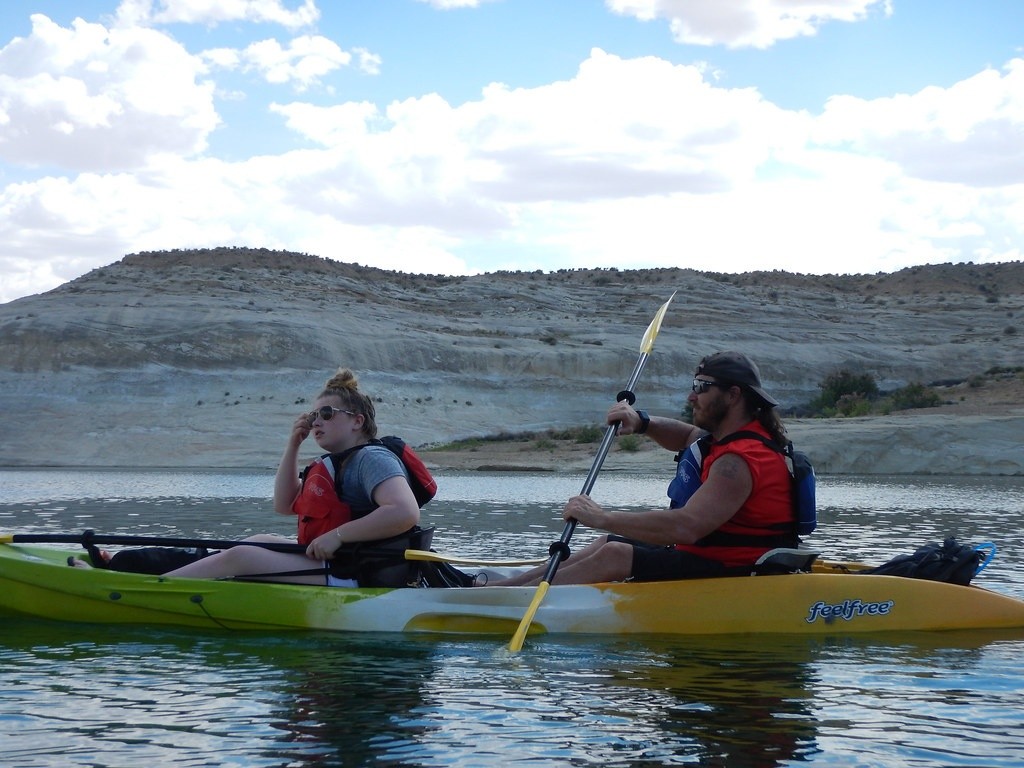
635;409;650;434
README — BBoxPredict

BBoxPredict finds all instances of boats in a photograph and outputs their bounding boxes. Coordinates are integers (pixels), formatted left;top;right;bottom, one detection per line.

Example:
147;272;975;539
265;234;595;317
0;531;1024;641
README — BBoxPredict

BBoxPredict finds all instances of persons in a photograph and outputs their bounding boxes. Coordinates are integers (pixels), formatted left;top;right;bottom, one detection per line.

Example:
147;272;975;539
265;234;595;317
75;367;425;591
424;349;800;591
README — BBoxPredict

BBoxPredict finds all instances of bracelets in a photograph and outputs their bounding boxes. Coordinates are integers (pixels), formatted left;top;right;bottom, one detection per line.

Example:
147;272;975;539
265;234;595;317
337;527;344;540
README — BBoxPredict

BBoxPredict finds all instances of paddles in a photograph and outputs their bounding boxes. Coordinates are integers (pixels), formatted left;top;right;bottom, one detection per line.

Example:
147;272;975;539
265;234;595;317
2;527;551;567
508;288;680;651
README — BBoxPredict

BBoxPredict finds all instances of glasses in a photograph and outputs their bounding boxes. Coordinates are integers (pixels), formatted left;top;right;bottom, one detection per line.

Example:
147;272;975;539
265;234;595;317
692;378;723;394
308;405;358;427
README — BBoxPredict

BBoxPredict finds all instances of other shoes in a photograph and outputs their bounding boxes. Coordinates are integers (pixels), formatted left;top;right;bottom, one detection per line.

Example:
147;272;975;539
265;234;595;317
67;555;78;567
420;549;488;588
90;546;109;567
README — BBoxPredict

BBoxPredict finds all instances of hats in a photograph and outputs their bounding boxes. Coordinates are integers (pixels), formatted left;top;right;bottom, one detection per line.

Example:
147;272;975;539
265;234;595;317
697;351;779;409
